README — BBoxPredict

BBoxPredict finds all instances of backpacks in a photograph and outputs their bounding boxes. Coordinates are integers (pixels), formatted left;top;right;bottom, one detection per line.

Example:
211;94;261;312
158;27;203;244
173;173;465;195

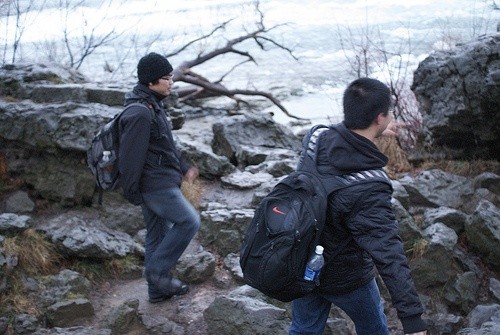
238;125;394;302
87;103;155;205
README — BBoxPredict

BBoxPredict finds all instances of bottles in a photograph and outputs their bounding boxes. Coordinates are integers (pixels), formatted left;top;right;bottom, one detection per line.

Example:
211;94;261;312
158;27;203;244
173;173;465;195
103;151;112;181
303;245;325;290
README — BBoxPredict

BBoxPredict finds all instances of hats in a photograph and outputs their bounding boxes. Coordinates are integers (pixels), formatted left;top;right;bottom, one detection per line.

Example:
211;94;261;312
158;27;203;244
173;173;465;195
137;51;173;83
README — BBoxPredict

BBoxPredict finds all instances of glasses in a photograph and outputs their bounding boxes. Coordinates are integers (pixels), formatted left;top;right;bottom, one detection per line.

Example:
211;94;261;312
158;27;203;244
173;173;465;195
384;103;395;114
158;72;174;80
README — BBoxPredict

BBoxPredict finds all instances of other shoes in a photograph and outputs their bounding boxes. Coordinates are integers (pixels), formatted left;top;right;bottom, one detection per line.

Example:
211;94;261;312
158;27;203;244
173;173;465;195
152;280;189;296
148;290;173;303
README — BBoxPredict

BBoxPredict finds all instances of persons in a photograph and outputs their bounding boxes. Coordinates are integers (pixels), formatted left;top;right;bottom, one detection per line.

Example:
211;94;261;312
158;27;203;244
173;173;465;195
116;53;201;304
290;78;429;335
